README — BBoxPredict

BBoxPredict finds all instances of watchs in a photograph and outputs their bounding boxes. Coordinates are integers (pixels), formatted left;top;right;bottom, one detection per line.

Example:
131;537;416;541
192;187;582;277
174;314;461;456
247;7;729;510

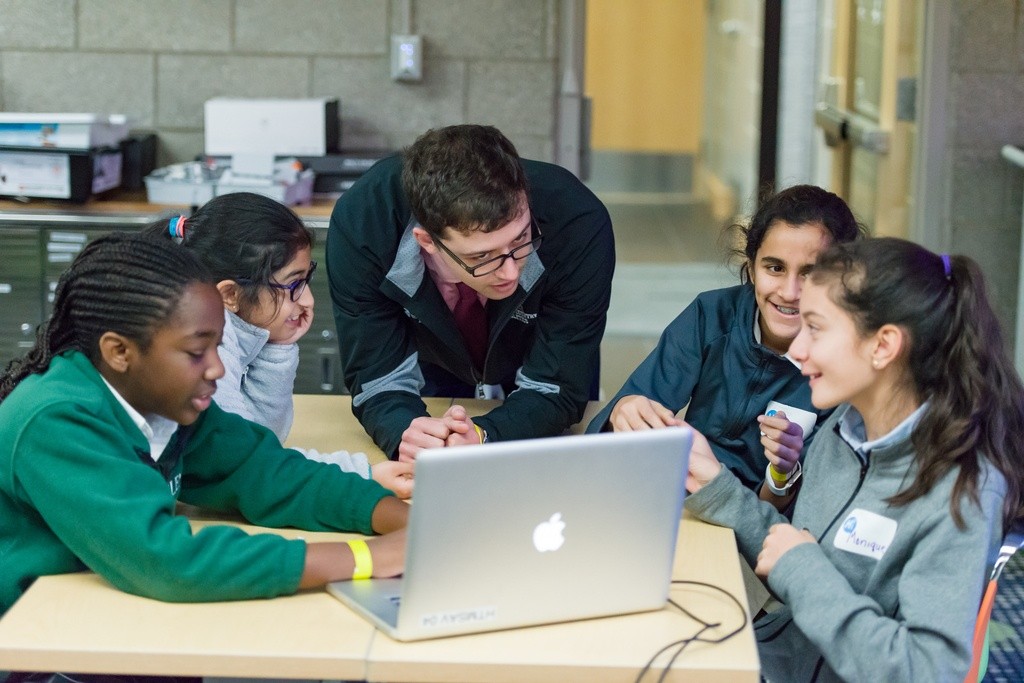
766;459;802;497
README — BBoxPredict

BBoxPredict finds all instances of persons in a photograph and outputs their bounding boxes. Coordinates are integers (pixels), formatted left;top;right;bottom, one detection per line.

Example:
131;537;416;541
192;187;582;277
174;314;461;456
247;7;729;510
0;230;410;683
676;237;1024;683
325;124;616;463
586;185;873;515
143;192;415;498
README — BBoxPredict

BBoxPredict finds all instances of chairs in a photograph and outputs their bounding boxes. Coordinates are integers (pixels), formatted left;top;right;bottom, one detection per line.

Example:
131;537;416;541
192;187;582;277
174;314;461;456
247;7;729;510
960;528;1024;683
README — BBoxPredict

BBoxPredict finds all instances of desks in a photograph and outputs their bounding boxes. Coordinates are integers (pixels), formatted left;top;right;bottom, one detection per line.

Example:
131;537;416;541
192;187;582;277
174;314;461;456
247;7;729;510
0;392;765;683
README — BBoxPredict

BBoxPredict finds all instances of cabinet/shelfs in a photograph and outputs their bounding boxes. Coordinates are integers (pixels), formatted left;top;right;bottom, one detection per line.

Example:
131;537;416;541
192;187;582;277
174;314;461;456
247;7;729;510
0;195;344;397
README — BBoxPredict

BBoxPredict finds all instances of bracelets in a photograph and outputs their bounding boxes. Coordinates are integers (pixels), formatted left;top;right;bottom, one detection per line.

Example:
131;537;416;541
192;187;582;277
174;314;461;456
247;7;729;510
474;424;482;444
346;539;373;580
770;462;797;481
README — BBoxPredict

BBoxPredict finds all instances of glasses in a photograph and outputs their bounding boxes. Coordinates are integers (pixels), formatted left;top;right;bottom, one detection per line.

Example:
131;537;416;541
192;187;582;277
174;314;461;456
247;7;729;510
424;212;543;277
216;261;318;303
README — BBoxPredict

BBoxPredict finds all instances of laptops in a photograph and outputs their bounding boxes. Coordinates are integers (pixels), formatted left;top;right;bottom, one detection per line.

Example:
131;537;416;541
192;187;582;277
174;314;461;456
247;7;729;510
327;427;694;642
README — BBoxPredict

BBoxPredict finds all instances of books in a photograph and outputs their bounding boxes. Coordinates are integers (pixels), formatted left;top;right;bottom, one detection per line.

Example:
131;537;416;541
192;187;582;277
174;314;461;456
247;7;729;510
0;110;128;148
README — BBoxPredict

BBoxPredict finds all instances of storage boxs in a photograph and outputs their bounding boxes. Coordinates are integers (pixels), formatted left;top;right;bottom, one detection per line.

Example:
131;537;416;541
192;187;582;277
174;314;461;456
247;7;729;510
1;110;315;216
